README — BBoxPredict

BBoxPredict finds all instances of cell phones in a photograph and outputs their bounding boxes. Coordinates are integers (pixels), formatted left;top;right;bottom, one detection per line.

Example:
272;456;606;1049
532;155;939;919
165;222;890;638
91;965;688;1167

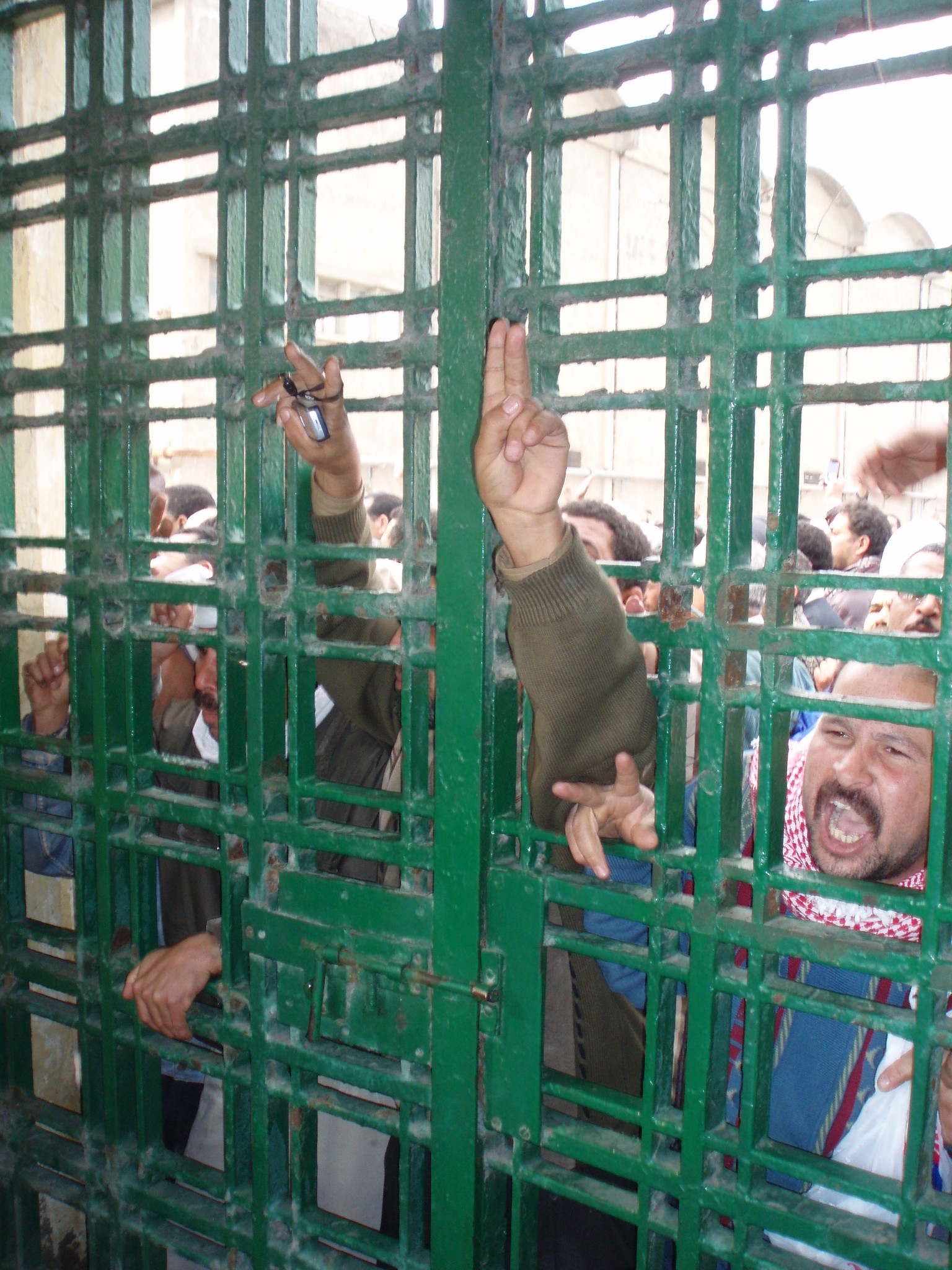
291;399;330;441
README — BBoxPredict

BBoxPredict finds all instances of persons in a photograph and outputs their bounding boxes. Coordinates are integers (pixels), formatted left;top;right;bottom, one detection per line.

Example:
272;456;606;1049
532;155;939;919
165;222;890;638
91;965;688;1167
19;316;952;1270
